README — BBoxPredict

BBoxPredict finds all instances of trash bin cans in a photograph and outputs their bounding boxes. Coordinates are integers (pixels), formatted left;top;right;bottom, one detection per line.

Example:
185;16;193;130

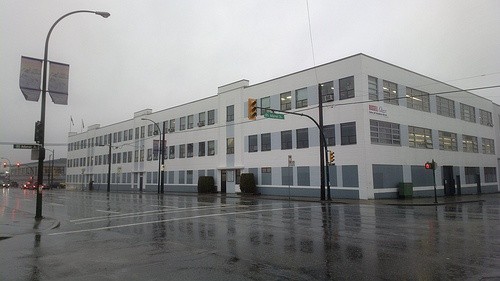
398;183;413;199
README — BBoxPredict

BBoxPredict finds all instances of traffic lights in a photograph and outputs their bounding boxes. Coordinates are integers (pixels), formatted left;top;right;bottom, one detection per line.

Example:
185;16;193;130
247;98;257;120
4;162;6;168
329;151;335;162
425;163;432;169
17;163;20;167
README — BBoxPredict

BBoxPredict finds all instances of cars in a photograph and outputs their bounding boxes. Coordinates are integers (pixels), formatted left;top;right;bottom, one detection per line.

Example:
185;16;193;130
2;181;19;188
20;182;66;190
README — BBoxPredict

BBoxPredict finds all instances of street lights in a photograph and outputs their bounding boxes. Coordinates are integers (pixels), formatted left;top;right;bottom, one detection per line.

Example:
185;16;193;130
35;10;111;219
141;117;161;195
